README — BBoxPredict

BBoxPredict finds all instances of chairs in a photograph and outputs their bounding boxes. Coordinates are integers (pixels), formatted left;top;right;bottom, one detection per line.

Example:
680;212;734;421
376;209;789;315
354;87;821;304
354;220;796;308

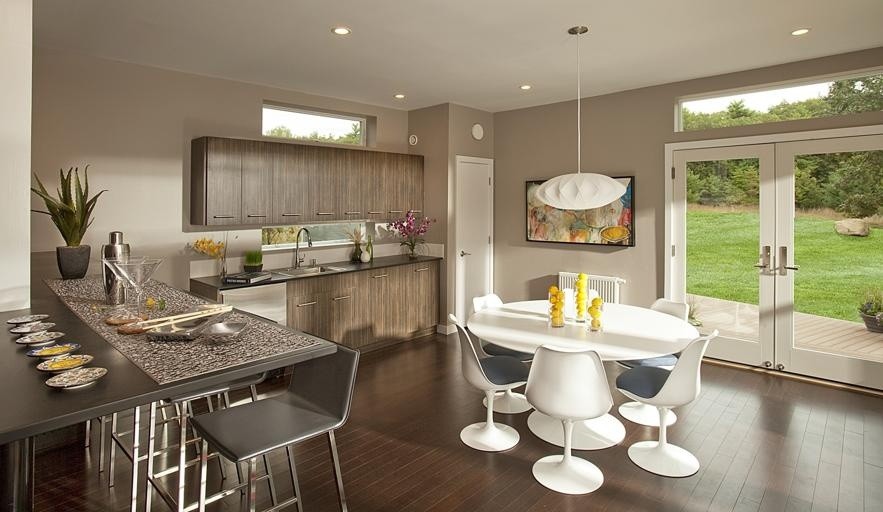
84;290;218;472
108;288;222;512
474;295;536;414
616;330;720;479
448;313;529;453
526;346;614;496
191;331;360;512
618;299;689;427
146;308;280;512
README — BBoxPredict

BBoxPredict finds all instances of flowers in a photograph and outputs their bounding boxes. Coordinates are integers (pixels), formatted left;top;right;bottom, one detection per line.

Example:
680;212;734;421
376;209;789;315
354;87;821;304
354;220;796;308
386;210;432;262
342;223;364;242
187;239;230;281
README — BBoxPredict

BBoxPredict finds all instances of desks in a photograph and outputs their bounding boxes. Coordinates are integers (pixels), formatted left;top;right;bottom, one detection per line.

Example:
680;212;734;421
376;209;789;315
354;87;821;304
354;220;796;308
469;300;702;452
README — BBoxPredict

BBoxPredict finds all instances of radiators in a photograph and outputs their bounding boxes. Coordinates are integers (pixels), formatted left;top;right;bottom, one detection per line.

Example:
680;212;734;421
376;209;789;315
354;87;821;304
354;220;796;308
559;272;626;304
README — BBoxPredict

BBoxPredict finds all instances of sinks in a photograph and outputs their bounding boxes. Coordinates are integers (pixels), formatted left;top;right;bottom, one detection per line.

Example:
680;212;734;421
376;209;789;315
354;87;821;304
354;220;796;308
271;267;335;277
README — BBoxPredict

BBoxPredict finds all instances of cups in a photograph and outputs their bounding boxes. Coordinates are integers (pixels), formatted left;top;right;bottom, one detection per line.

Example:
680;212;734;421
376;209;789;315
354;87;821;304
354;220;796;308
117;257;165;329
101;257;151;320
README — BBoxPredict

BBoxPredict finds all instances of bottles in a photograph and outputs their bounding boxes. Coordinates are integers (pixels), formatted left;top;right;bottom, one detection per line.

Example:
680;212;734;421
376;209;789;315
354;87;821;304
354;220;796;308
366;235;374;261
101;232;130;306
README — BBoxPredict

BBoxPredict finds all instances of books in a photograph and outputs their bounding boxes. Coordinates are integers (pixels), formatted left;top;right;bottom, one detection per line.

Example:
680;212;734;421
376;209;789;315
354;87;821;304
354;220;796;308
225;271;272;284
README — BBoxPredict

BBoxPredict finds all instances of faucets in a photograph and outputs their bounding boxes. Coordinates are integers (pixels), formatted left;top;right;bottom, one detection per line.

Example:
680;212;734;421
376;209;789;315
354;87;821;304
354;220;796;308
295;227;313;268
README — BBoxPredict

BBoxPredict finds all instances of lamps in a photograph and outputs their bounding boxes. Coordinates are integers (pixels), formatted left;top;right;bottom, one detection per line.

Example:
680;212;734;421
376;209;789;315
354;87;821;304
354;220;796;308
535;26;626;210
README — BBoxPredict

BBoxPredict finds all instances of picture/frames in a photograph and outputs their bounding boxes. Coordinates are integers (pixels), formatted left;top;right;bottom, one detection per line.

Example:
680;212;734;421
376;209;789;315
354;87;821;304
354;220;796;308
526;176;635;247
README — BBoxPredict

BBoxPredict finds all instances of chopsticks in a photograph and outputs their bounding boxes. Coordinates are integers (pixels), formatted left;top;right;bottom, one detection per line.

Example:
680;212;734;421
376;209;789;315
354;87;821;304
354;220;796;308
135;304;233;331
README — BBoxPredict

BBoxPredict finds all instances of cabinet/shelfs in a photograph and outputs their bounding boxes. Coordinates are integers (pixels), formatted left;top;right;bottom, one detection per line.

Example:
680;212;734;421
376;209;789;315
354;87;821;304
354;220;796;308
372;255;444;348
286;259;370;350
190;253;287;375
191;138;273;226
274;144;338;224
386;154;425;221
339;149;385;221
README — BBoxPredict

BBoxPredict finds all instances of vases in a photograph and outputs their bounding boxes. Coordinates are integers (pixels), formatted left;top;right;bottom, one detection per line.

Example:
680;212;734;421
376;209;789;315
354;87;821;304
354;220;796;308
409;246;418;259
353;243;362;262
219;261;227;283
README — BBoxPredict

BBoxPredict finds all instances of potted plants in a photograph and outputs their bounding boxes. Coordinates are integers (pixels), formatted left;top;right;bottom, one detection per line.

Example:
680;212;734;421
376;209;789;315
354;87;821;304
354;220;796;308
31;165;110;278
243;249;263;272
688;293;704;337
858;295;883;334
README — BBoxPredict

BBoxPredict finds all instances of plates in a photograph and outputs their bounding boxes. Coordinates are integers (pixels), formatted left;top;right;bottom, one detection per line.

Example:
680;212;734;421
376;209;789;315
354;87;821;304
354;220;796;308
6;314;50;326
7;321;58;336
25;340;84;359
200;322;247;346
36;355;94;374
44;366;108;391
15;331;67;347
